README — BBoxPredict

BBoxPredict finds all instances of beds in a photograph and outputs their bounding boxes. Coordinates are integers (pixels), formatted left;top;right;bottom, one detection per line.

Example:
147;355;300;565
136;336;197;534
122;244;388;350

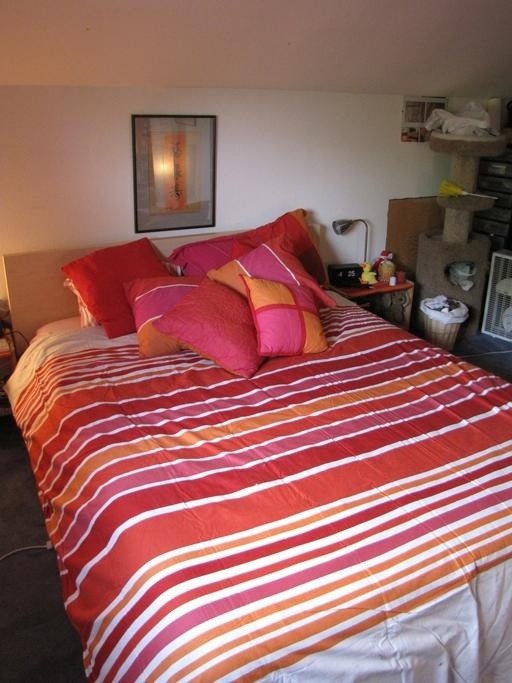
2;222;512;683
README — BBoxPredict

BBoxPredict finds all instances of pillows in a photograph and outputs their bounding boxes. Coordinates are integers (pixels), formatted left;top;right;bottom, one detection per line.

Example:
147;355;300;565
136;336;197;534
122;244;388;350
122;275;207;361
63;261;185;329
168;208;326;288
207;233;337;310
59;237;174;339
239;273;328;357
151;276;266;378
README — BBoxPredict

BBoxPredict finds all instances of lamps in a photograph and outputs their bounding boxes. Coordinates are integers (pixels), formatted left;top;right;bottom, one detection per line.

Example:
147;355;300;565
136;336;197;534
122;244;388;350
332;219;369;264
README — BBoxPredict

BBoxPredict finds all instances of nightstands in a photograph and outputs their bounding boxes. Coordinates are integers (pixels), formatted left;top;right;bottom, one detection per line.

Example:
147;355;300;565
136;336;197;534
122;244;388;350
0;327;18;447
335;279;415;331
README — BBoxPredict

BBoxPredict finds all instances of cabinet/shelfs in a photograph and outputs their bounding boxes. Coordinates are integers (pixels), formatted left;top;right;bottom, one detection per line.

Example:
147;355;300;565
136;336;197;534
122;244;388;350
473;155;512;253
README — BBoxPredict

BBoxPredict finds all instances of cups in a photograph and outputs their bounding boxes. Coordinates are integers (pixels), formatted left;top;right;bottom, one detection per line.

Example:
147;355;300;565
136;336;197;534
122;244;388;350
395;271;406;284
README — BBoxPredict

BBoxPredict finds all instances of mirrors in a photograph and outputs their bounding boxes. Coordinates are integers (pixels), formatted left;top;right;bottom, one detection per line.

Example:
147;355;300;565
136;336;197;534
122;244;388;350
131;114;218;234
